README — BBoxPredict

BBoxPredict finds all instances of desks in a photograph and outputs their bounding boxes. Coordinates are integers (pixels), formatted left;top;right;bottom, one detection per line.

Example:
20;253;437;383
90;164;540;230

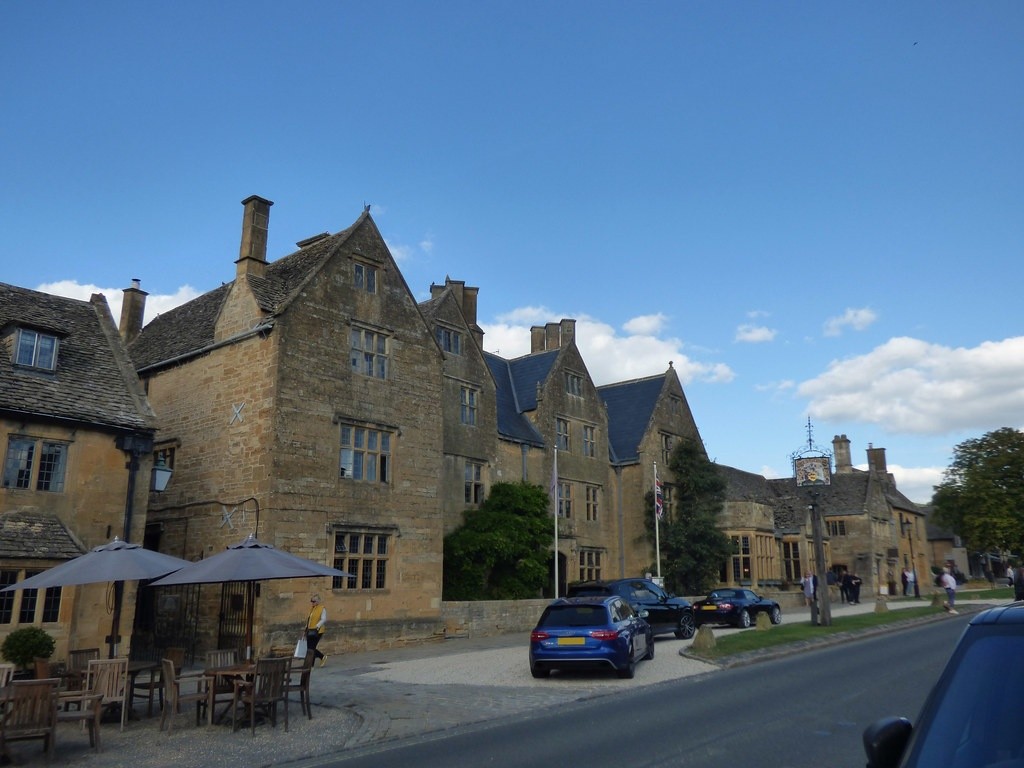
77;661;158;721
204;665;257;726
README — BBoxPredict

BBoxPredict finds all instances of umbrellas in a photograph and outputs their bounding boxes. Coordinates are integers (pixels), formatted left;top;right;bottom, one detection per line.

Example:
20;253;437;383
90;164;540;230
146;534;357;663
1;536;195;660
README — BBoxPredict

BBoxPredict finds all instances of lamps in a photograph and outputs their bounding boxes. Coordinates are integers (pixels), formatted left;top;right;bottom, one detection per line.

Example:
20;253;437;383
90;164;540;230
150;450;174;492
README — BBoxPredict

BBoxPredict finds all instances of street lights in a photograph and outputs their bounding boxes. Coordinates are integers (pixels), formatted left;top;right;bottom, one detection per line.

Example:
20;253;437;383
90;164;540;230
902;517;920;599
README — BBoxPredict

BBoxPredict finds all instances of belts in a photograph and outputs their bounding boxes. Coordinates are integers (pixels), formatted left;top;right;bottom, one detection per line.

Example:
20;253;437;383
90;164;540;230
945;588;951;590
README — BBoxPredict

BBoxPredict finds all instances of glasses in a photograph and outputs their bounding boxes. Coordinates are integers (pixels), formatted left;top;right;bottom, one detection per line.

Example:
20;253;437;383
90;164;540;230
311;600;316;603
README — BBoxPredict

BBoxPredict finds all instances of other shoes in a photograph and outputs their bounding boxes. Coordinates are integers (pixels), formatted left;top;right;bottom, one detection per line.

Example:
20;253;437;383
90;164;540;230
944;600;959;615
1004;584;1010;588
904;594;910;597
850;600;861;605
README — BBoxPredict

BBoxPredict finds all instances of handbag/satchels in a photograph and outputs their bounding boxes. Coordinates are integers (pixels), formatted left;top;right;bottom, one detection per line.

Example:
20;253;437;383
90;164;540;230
293;636;308;659
800;584;805;591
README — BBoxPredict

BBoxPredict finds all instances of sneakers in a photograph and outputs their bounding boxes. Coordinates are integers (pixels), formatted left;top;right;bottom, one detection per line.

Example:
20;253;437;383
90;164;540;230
311;667;317;671
320;655;328;667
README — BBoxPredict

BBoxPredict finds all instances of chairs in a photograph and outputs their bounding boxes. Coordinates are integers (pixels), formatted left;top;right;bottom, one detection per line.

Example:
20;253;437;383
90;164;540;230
0;657;130;765
135;645;314;736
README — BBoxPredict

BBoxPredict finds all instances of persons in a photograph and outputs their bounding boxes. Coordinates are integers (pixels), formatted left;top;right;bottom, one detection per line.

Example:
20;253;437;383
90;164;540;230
940;567;959;614
901;567;914;595
304;595;328;672
837;569;862;604
800;570;819;607
1004;565;1014;588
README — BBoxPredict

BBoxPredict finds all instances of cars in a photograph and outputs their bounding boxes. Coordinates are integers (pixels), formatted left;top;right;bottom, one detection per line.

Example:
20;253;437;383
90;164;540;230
692;587;782;629
567;578;696;639
529;596;654;679
862;600;1024;768
